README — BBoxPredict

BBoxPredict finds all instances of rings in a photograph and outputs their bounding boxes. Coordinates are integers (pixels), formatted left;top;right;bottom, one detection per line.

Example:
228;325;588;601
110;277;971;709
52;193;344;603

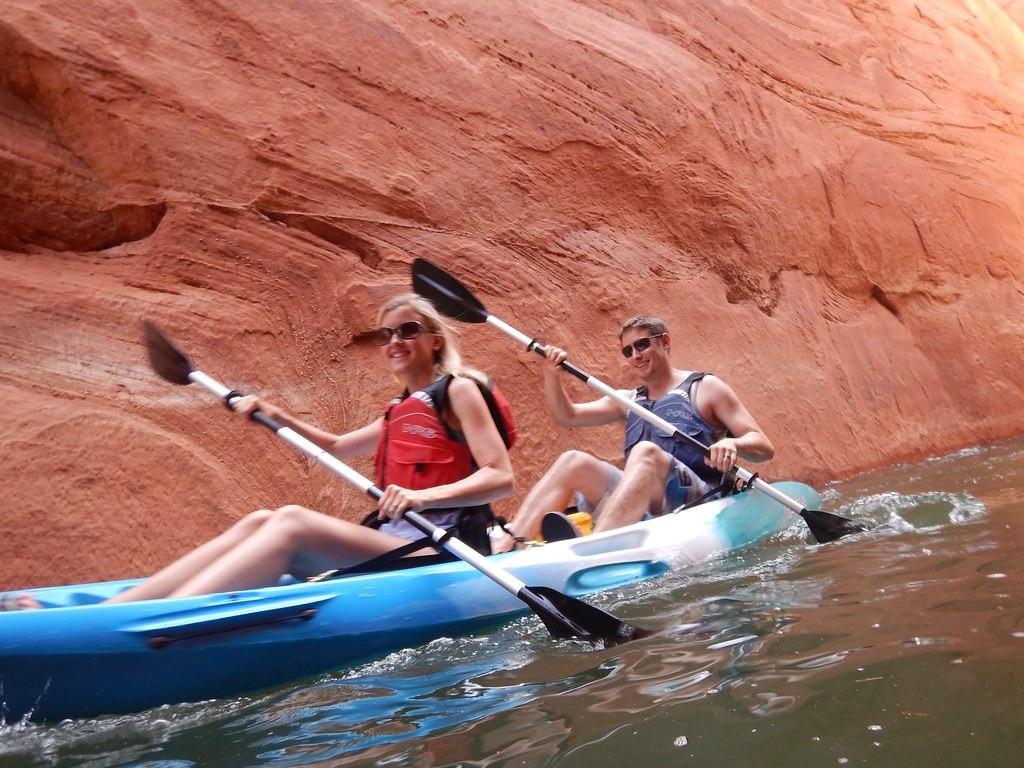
393;503;398;509
724;455;731;459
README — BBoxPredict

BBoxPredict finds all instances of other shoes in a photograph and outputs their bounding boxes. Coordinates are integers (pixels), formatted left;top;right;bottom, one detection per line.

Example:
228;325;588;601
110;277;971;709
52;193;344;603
1;593;45;612
540;512;580;543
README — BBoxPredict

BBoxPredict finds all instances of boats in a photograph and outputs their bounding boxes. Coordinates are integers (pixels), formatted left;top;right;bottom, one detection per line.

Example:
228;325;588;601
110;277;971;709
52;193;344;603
0;480;822;725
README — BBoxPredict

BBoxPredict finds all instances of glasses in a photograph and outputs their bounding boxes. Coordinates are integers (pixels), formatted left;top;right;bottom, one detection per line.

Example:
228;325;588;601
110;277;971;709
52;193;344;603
622;333;664;358
374;320;436;346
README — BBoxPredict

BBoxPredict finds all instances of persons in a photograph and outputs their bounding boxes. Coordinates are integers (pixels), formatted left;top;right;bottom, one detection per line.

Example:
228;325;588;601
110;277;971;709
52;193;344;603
488;314;775;554
0;293;516;610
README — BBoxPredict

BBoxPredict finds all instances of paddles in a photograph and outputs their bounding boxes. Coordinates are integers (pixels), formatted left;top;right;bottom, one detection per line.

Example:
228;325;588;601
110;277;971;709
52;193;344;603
141;318;633;643
410;256;870;546
